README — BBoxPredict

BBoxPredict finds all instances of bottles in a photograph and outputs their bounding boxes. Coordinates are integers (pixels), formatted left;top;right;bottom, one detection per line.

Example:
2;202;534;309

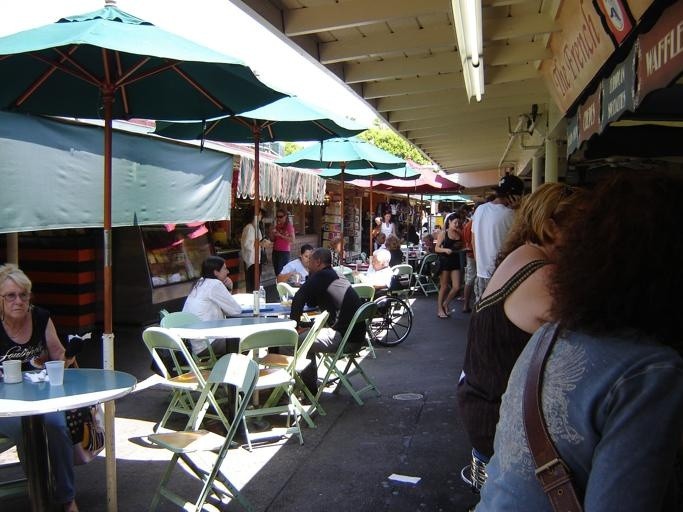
259;285;267;305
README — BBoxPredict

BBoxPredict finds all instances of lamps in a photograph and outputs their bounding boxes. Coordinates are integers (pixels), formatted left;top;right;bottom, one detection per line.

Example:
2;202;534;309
449;0;483;66
460;55;485;106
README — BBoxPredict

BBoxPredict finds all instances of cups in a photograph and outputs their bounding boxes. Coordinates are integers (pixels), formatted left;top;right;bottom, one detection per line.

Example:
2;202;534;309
3;359;23;385
355;260;362;268
44;360;65;385
291;274;302;285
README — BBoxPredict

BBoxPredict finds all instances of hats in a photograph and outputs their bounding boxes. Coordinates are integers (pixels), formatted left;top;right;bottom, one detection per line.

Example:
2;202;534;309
490;174;525;197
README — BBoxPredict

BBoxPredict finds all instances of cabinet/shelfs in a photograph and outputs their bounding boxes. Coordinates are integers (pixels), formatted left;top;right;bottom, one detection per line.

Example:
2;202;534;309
109;223;217;331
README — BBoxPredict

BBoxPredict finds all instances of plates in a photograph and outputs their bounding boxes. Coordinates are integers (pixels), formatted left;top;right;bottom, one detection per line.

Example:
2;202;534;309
280;304;291;307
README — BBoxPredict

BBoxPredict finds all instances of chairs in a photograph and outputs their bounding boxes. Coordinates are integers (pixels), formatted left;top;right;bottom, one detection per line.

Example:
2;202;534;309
391;244;439;298
275;278;382;406
143;294;329;447
148;353;260;511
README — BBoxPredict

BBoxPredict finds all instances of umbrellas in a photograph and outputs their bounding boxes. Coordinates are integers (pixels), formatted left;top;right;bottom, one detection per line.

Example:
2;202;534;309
0;1;293;512
275;139;406;268
149;99;368;404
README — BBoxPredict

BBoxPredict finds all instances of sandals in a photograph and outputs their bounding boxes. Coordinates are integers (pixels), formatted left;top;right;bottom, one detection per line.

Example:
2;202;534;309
437;311;448;318
442;301;449;315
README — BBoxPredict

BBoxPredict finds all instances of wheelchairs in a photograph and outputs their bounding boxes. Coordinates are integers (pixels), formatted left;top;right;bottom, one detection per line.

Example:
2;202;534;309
358;267;414;347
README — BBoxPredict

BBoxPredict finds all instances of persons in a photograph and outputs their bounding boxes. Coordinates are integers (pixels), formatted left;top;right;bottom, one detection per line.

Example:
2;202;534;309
0;264;78;512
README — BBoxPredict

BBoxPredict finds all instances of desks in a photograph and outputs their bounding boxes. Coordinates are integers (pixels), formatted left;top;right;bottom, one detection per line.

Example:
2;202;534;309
0;368;138;511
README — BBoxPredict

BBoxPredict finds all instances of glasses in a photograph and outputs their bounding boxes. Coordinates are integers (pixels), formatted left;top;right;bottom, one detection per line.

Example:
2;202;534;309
0;292;31;302
276;214;286;219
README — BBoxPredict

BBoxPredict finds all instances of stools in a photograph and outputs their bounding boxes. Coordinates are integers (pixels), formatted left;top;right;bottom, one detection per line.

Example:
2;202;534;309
459;449;489;497
